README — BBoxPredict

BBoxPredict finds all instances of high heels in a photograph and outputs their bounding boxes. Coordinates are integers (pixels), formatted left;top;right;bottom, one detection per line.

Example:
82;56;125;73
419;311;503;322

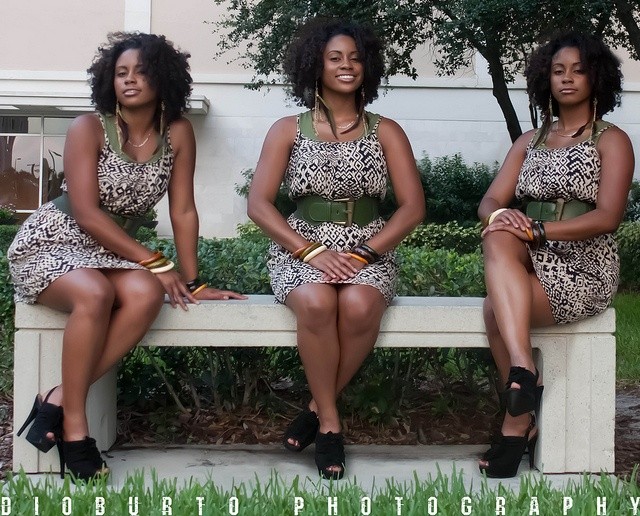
314;423;346;480
282;397;319;452
504;366;544;417
54;418;109;486
17;385;64;452
479;414;538;478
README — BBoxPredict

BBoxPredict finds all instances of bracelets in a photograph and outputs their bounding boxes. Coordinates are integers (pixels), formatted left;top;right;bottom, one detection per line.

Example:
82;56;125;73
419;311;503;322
303;245;327;263
538;223;546;240
526;227;534;241
485;213;491;226
532;223;540;243
192;283;208;295
186;279;201;287
347;253;368;266
292;242;314;259
149;260;175;273
361;243;379;261
488;208;508;225
353;247;375;264
139;252;162;264
299;242;322;260
145;257;169;268
188;284;201;292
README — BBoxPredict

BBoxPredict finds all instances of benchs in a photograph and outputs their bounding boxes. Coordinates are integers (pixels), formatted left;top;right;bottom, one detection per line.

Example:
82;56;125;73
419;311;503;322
12;294;617;474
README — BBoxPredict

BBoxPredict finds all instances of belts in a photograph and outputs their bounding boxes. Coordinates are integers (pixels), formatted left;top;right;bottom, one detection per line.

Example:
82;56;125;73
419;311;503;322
525;197;594;222
294;194;380;228
52;190;158;237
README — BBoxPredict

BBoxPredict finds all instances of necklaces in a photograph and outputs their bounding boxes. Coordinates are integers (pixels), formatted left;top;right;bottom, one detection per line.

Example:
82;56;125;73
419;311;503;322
127;122;156;148
556;121;577;138
319;110;360;129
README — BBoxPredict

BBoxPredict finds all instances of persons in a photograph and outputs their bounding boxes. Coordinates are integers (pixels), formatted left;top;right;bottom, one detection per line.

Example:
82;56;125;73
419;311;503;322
477;32;634;478
6;33;249;487
247;14;426;480
